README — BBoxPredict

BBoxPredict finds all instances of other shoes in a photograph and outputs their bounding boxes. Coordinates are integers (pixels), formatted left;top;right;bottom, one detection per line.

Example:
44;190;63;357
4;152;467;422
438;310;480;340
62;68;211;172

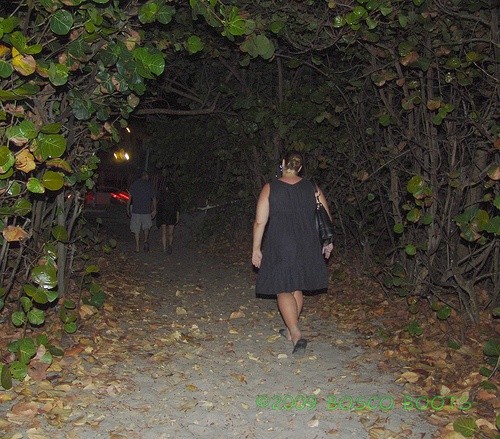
144;242;148;252
168;246;172;254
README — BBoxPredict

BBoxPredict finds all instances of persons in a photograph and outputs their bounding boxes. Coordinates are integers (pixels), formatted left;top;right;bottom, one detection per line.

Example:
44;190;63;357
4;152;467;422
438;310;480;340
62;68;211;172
251;150;334;356
156;182;180;256
126;171;157;253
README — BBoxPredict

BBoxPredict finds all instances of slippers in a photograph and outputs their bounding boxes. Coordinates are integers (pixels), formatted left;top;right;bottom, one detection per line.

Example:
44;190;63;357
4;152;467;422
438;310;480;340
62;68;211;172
292;339;307;355
279;329;292;341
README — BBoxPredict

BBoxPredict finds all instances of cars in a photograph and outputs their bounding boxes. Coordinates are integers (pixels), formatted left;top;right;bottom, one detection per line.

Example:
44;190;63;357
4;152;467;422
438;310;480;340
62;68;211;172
79;160;132;229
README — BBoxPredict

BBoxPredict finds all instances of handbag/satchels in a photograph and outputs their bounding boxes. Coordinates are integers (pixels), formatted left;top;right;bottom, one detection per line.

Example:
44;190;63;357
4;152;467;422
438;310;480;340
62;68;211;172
311;180;336;244
